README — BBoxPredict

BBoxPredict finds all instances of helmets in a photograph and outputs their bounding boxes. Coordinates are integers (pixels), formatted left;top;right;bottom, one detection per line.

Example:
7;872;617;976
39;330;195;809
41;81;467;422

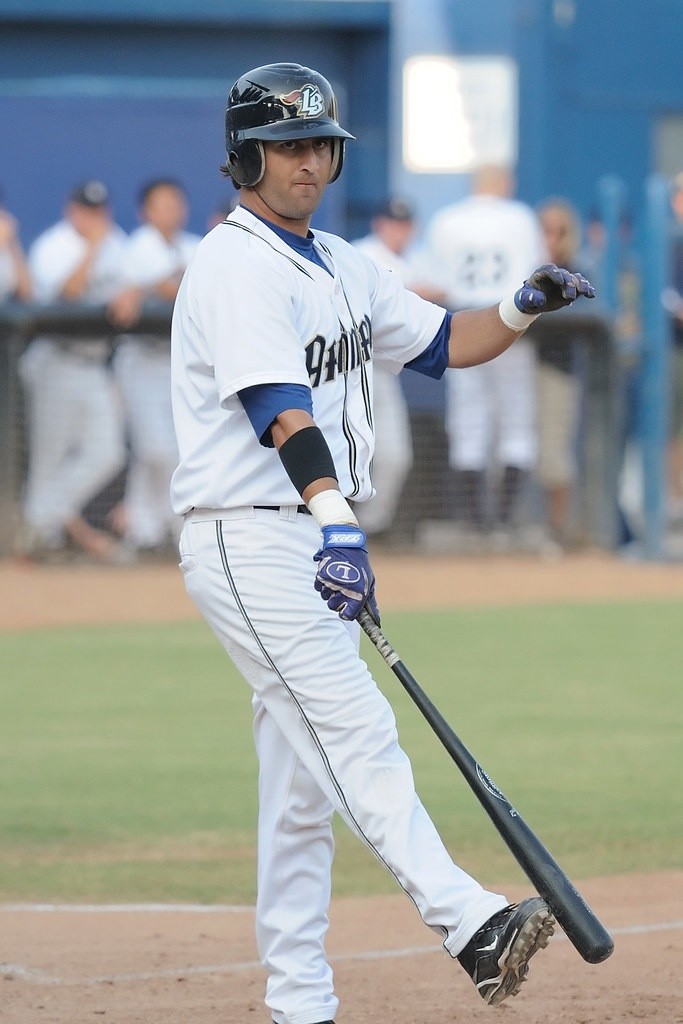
223;62;356;187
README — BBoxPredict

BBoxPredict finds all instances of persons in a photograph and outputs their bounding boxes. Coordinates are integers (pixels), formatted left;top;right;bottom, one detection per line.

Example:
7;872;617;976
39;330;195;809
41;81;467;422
0;161;682;564
168;64;595;1024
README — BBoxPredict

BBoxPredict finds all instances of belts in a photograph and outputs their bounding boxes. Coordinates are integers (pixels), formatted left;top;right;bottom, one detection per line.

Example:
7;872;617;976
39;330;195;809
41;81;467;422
253;498;358;515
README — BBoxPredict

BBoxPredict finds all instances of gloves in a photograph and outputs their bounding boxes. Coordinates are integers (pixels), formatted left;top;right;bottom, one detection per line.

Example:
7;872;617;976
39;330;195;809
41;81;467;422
499;264;596;330
314;523;387;630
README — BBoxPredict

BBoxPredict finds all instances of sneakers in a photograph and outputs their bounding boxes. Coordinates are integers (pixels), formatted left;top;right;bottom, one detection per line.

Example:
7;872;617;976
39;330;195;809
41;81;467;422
457;896;556;1009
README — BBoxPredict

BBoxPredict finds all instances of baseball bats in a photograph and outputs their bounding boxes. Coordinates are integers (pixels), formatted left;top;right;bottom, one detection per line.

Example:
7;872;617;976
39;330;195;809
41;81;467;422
356;605;614;964
